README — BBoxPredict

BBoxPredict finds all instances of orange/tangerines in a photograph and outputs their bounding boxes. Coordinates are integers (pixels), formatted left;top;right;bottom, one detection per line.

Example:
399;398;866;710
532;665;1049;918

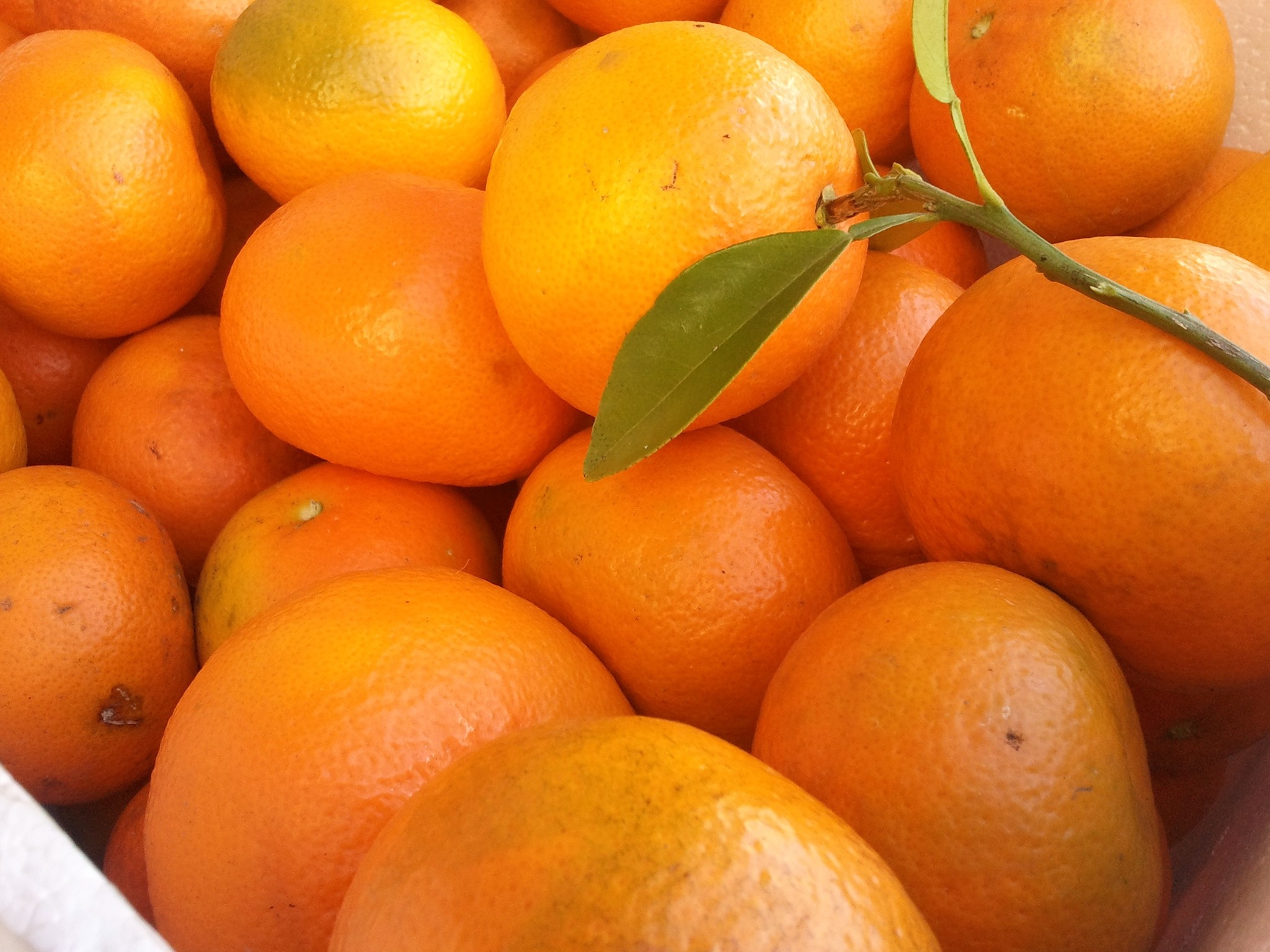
1;0;1270;952
